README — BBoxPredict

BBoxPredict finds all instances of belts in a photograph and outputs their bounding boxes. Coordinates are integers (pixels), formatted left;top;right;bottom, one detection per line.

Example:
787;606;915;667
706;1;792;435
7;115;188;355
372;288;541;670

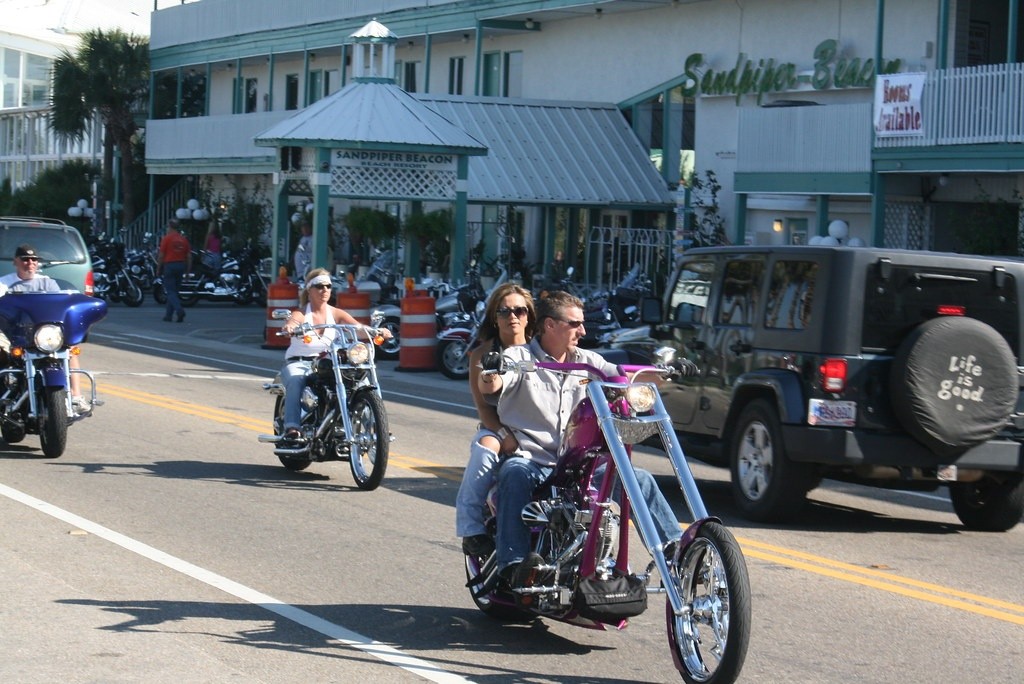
288;356;315;361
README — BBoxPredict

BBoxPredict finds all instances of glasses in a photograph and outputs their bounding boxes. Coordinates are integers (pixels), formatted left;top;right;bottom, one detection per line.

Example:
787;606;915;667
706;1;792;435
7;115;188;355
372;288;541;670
495;307;530;318
312;284;332;289
537;315;585;328
19;258;38;261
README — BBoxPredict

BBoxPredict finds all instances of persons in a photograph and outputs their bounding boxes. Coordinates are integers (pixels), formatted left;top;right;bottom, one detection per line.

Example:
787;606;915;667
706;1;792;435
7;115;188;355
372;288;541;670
294;218;313;281
201;222;222;270
156;221;191;322
456;283;538;556
281;269;392;439
0;243;93;417
478;291;710;591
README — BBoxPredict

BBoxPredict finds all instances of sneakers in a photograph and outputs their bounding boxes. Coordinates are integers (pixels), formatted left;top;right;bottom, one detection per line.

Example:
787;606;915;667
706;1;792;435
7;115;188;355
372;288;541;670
71;396;93;417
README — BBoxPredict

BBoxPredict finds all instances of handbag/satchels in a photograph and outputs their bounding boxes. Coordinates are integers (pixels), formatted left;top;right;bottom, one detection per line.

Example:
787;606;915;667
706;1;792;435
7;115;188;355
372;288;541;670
575;567;647;621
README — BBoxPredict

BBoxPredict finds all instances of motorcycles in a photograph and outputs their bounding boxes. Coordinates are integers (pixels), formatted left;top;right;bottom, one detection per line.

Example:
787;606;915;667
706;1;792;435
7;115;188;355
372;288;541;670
83;231;658;382
0;289;110;459
463;346;753;684
257;308;395;491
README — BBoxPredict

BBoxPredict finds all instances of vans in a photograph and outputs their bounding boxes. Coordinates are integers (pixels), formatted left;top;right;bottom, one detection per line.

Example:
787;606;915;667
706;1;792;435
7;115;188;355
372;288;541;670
0;216;96;345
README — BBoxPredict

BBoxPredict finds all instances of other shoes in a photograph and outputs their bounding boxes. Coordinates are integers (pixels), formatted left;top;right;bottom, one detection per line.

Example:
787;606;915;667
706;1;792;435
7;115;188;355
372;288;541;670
511;552;546;610
163;314;172;321
285;428;301;438
177;312;185;322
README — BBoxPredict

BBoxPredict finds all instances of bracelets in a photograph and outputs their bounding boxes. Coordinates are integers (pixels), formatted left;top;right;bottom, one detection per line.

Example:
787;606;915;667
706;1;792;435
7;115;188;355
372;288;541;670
482;374;497;383
497;427;509;440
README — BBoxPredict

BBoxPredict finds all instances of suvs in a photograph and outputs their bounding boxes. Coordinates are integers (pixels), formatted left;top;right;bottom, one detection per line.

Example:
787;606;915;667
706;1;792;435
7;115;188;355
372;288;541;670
608;246;1023;533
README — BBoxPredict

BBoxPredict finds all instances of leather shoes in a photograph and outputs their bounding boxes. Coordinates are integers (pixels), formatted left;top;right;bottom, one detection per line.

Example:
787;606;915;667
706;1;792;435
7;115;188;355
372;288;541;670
462;535;495;555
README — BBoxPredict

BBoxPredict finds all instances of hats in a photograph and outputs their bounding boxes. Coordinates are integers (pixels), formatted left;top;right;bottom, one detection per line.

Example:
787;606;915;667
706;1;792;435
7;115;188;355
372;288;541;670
170;219;180;228
16;245;37;257
